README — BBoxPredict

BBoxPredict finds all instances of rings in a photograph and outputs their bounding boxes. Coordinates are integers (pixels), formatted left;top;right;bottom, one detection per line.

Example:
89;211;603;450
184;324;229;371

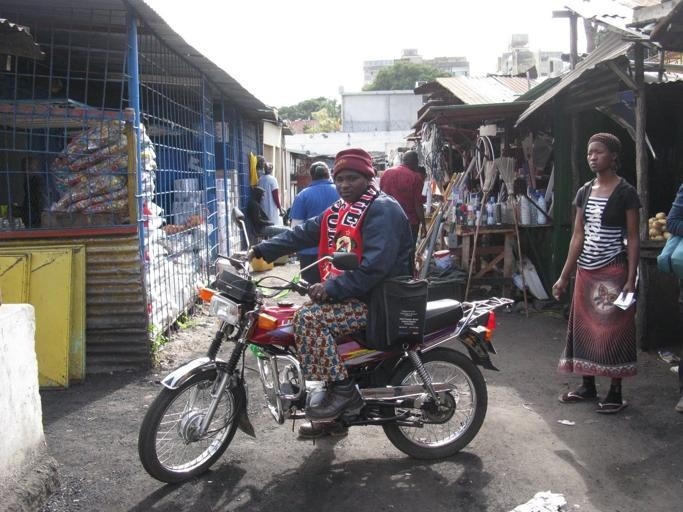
316;292;321;297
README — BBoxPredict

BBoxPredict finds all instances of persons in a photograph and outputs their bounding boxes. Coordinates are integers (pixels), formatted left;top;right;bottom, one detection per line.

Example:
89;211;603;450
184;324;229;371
377;149;427;279
415;163;427;183
256;162;287;229
287;161;342;285
9;154;48;228
658;184;681;415
228;147;417;438
552;132;641;413
248;186;300;264
372;162;389;193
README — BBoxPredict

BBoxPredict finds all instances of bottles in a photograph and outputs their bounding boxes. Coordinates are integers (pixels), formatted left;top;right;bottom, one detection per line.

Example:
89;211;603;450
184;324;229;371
455;192;550;227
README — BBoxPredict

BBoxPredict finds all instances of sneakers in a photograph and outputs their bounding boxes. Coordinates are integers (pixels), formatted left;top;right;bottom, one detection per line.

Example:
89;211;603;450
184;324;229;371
675;396;683;412
309;376;363;417
298;421;348;439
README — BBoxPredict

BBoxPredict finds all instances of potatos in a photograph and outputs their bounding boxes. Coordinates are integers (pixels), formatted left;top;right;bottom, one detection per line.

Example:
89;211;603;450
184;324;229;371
648;212;673;242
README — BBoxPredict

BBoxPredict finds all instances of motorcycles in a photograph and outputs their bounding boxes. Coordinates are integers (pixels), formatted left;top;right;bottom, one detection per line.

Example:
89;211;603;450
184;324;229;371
136;207;516;486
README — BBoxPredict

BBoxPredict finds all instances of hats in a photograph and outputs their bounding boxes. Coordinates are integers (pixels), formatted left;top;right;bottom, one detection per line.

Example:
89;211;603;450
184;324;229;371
587;133;622;152
264;162;273;172
332;149;375;177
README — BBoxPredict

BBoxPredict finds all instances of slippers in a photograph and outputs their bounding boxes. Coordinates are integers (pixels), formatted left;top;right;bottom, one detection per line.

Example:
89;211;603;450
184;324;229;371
595;400;628;413
559;391;600;403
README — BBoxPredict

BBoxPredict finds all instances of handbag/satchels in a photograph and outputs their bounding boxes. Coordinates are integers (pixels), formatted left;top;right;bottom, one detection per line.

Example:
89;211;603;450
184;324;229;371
657;234;683;278
365;277;429;349
